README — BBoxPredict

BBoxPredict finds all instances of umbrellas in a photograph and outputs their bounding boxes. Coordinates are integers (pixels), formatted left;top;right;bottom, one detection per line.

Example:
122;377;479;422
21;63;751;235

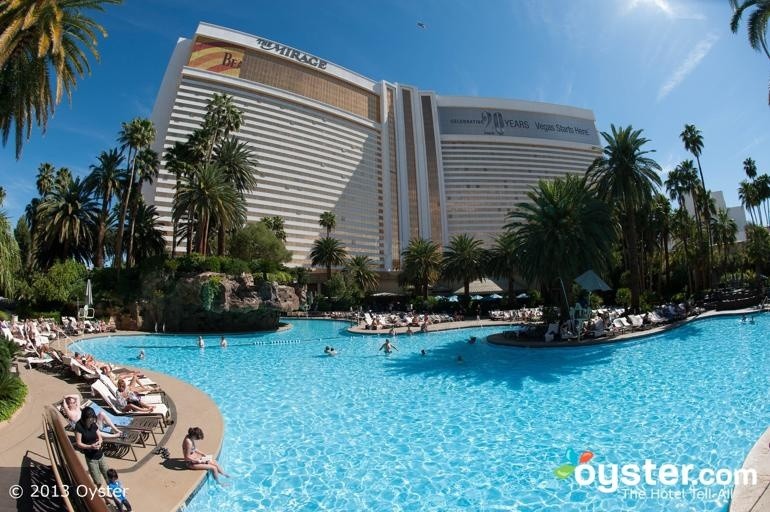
85;279;93;306
573;270;612;304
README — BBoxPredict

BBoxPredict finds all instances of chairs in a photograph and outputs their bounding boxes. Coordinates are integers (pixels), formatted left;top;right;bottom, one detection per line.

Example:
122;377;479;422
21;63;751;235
0;316;170;461
325;299;704;340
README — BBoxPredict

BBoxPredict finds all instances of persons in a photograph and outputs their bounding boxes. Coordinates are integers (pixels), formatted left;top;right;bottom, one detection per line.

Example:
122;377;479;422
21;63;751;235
107;469;132;512
220;336;227;347
63;394;123;438
199;336;204;348
0;315;160;412
75;407;110;505
183;427;230;484
325;295;694;357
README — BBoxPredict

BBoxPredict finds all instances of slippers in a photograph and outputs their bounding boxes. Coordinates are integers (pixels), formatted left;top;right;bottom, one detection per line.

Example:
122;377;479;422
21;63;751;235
155;447;170;458
